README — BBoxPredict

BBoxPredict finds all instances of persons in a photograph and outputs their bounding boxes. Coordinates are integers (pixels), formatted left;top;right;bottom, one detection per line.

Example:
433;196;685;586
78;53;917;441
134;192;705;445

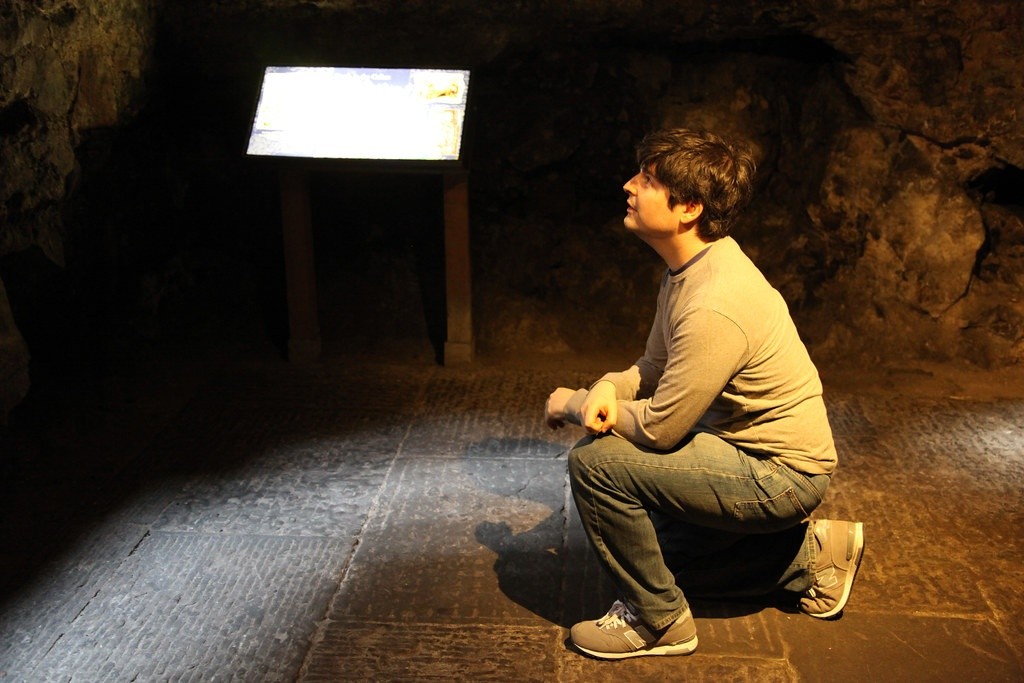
542;126;864;660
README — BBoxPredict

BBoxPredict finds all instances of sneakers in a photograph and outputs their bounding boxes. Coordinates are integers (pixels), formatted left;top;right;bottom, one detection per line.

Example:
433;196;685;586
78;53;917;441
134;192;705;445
795;519;867;623
567;595;703;660
